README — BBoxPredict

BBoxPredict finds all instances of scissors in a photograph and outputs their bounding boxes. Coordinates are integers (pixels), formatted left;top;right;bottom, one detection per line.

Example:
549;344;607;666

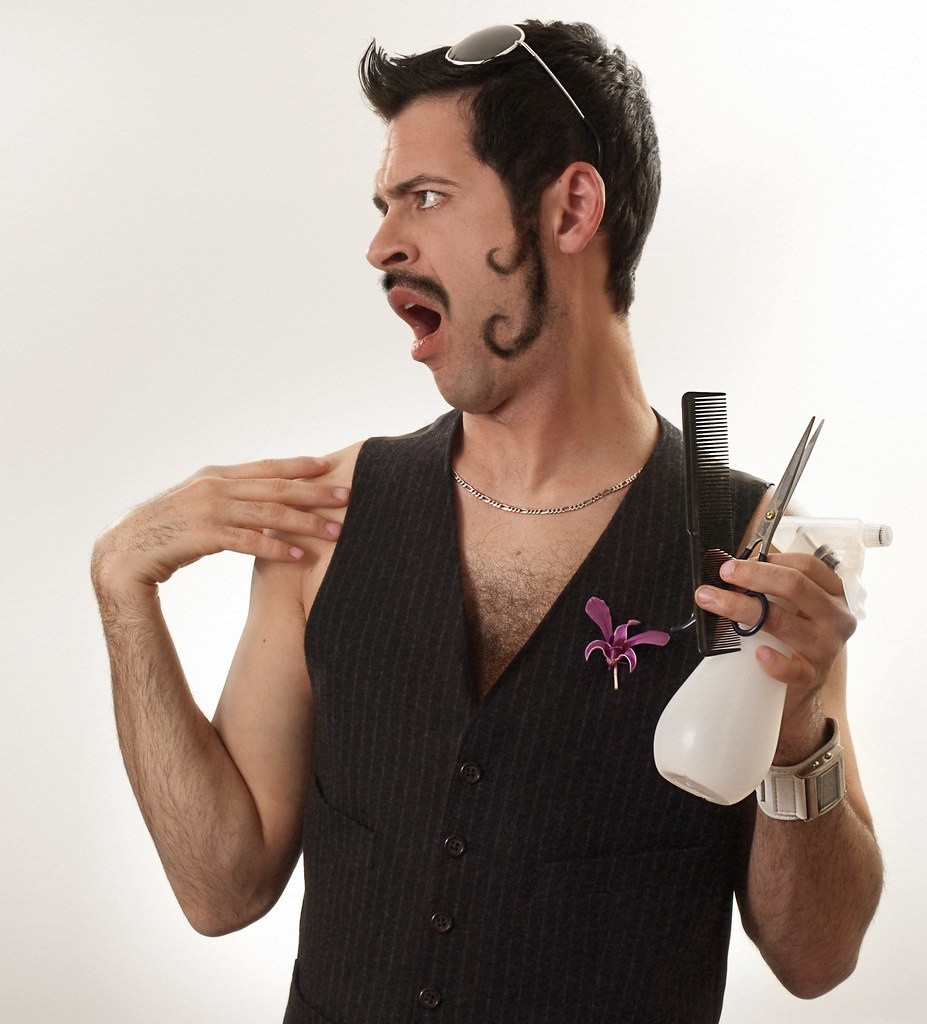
669;415;825;639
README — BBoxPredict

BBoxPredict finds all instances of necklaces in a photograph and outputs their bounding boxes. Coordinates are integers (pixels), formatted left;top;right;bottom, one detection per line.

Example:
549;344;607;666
450;466;643;514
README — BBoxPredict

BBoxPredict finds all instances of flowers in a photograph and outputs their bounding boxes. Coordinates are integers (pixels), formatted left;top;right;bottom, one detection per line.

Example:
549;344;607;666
585;596;671;690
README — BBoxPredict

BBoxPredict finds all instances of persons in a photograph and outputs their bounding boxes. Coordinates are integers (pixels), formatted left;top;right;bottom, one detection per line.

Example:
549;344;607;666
88;18;882;1024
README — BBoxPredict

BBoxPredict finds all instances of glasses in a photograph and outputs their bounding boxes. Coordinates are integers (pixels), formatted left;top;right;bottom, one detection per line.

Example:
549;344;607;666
445;24;603;177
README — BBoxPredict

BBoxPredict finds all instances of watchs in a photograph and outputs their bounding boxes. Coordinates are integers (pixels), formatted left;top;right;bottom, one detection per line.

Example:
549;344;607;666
756;717;847;822
